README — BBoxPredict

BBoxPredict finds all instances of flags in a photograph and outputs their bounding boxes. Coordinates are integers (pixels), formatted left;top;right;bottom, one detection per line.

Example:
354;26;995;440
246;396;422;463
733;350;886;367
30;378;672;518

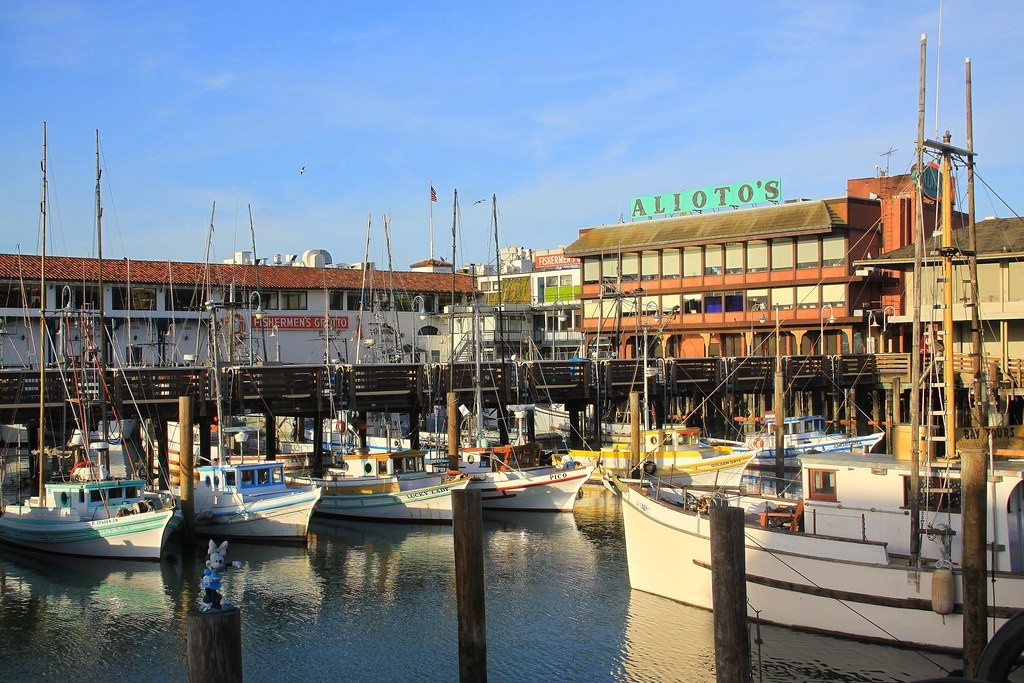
431;187;437;202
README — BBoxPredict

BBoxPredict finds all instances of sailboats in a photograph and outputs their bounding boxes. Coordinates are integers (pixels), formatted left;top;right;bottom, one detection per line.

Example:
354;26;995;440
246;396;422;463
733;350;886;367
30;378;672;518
593;1;1024;660
0;116;886;560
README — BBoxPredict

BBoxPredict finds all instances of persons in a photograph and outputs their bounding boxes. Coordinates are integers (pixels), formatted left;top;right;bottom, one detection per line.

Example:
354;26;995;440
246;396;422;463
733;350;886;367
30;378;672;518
322;353;332;364
511;354;518;362
609;353;618;359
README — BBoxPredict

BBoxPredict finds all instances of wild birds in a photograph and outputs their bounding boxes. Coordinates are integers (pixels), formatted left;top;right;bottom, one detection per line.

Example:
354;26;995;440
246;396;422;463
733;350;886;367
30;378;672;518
473;198;486;205
300;166;306;175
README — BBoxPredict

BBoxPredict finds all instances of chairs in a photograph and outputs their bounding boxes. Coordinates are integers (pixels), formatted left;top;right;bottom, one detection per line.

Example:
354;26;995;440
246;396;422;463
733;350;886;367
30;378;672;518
757;498;803;530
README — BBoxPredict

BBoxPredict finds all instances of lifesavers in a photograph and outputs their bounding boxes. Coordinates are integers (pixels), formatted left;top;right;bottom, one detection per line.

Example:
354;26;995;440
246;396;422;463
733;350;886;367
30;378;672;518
643;461;656;476
336;420;345;434
70;461;95;474
752;438;764;448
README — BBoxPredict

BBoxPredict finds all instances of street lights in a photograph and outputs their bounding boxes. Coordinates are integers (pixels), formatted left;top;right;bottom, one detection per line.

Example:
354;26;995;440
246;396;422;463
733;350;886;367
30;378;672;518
248;291;270;366
820;304;838;356
412;296;431;387
868;314;880;354
751;303;770;376
645;299;663;324
58;284;82;369
551;301;571;383
